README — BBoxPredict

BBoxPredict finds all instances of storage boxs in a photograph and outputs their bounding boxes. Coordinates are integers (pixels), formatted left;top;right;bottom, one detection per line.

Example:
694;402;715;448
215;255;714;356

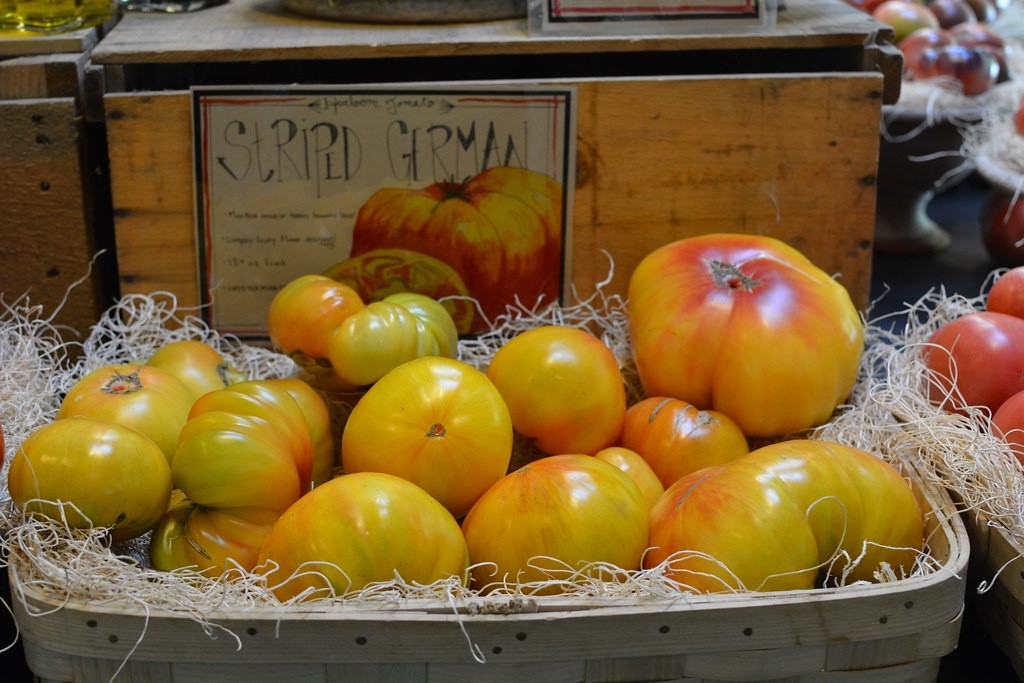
5;388;968;682
91;0;902;347
893;410;1024;682
0;25;104;371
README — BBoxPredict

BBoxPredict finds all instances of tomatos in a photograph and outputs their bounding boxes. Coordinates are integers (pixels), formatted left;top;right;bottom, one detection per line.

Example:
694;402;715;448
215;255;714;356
916;265;1024;474
840;0;1024;133
0;231;921;595
